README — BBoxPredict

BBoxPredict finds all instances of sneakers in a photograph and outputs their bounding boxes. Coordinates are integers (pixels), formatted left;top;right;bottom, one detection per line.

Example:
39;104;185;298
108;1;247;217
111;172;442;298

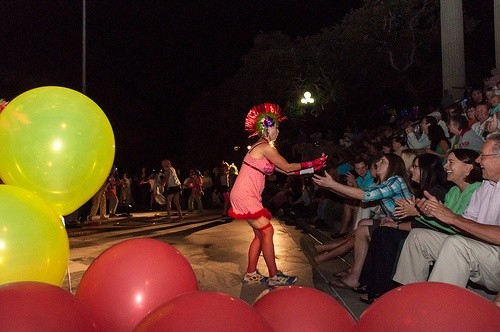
242;269;269;284
266;270;299;288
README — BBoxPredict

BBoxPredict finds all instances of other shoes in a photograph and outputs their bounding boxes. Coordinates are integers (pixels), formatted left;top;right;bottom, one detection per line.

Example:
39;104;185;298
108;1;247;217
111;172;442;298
173;217;184;223
100;216;108;221
304;251;319;267
331;231;348;239
353;285;370;294
187;209;193;213
361;293;382;305
160;217;171;223
87;216;92;221
300;235;317;257
109;214;118;218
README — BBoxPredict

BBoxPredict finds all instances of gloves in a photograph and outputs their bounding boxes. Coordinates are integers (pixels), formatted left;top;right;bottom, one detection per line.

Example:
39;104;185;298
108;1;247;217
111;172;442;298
300;153;328;170
294;160;327;176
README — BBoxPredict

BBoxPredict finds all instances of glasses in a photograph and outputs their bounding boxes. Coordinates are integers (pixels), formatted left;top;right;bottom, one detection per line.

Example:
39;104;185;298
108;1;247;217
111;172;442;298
479;154;497;159
411;164;419;169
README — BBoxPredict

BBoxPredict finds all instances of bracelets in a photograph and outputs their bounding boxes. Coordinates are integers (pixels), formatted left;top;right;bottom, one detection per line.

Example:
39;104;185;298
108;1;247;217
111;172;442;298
396;222;403;230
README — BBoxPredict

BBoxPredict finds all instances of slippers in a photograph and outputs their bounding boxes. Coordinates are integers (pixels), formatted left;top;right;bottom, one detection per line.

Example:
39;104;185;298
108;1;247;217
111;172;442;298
333;270;351;278
329;278;359;288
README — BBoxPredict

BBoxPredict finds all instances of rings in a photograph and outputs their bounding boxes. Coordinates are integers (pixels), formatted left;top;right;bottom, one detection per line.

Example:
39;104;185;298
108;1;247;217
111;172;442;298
403;213;405;215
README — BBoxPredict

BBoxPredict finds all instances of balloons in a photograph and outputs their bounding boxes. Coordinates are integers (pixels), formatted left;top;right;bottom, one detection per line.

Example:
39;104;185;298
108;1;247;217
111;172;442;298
132;290;274;332
75;238;198;332
0;281;95;332
253;285;357;332
0;86;116;217
0;185;70;285
357;281;500;332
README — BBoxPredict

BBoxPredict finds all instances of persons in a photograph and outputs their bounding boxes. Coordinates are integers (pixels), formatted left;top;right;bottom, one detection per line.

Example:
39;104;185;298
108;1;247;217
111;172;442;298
263;68;500;309
138;159;239;224
74;168;132;224
228;103;328;289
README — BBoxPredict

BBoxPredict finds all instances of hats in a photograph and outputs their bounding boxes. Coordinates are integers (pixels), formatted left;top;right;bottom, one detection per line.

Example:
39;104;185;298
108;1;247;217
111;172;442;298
245;103;288;139
427;110;442;120
